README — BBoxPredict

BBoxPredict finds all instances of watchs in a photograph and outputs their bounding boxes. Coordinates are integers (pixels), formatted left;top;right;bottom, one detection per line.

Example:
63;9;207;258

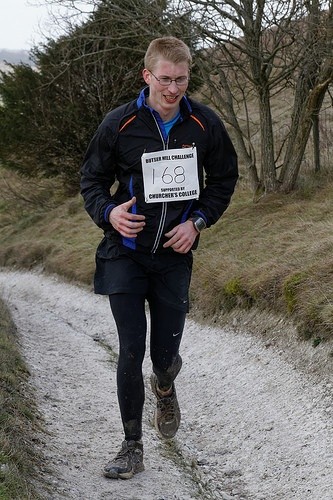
188;214;207;233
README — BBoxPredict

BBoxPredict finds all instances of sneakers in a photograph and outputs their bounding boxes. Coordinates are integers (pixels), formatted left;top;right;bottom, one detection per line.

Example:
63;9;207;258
149;373;181;439
103;439;146;480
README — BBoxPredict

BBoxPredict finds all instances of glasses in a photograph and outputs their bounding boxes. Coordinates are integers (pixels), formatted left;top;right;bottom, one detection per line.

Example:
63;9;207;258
148;70;190;86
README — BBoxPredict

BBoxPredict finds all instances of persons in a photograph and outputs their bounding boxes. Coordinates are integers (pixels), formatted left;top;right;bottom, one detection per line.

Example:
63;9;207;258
79;36;240;479
